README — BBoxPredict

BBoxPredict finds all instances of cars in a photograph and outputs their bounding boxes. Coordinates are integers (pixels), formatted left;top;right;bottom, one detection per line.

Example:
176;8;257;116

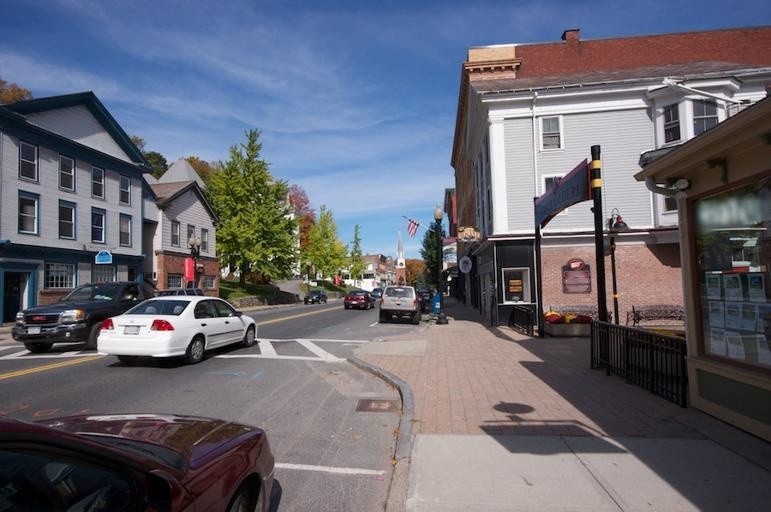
344;290;377;309
372;287;382;298
303;290;327;305
0;408;275;512
95;295;257;364
417;288;432;312
155;288;206;298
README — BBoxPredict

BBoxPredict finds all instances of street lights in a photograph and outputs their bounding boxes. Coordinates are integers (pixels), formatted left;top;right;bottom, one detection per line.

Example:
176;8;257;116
188;236;201;287
606;207;629;325
433;203;449;325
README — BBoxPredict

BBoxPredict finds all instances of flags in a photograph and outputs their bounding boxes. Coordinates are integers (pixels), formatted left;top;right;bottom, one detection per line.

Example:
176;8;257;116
407;218;419;238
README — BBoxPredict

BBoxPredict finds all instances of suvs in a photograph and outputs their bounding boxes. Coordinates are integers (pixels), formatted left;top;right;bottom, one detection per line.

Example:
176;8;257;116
12;281;149;353
379;285;421;324
